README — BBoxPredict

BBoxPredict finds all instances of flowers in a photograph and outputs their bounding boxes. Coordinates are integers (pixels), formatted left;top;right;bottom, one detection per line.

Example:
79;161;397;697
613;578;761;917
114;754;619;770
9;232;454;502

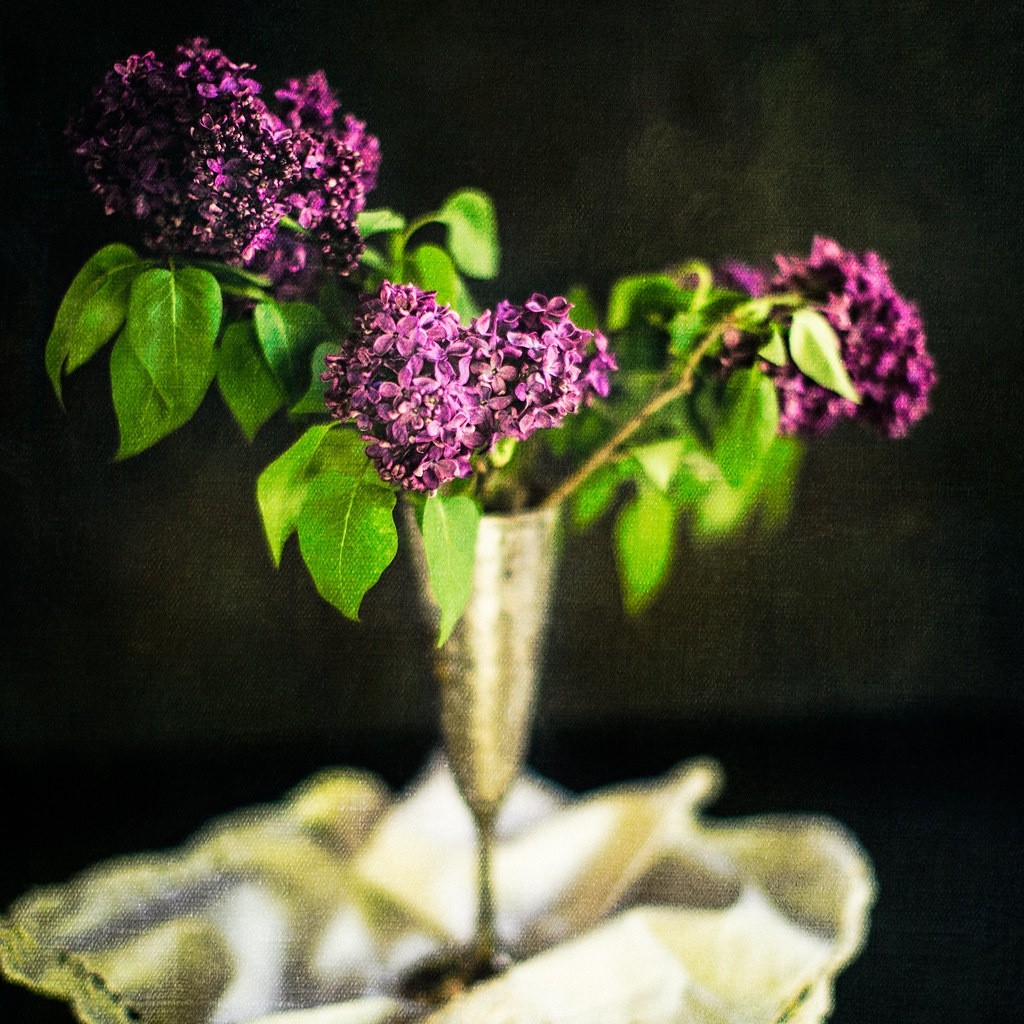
43;36;937;623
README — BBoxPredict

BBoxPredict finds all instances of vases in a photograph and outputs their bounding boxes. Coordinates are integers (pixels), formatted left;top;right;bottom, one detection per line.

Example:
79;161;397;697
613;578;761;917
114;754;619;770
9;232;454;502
403;508;562;959
